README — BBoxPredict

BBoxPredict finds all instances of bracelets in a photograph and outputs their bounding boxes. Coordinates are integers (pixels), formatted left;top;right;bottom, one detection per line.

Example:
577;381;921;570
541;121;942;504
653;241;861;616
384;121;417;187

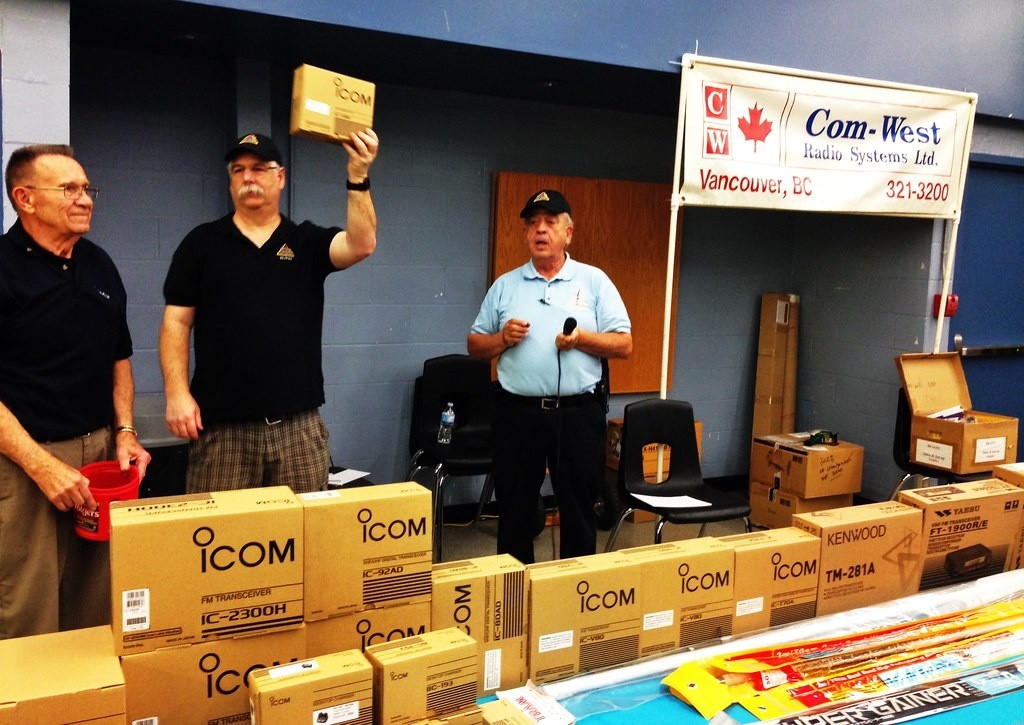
116;425;138;436
347;176;370;192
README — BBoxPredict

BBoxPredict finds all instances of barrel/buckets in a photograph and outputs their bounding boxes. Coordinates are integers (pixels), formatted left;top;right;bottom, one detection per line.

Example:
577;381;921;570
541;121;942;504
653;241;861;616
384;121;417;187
73;461;141;541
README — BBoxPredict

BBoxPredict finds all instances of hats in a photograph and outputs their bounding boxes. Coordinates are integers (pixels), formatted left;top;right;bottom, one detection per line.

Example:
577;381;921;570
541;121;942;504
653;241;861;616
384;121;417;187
223;133;289;167
520;189;572;221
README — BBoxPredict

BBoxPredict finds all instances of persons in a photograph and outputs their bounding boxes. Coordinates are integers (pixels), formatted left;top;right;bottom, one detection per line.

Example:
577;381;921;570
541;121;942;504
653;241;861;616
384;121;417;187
158;125;377;494
467;189;633;567
0;143;151;641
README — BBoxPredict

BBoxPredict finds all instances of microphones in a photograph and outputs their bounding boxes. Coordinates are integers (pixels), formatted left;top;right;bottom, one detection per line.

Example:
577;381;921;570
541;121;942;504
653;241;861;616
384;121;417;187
563;318;577;336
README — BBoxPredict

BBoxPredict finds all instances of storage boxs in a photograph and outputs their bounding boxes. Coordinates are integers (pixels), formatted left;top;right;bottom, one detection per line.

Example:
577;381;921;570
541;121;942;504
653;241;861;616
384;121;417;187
305;601;431;661
750;431;864;499
0;625;126;725
894;352;1018;475
110;485;304;655
248;648;373;725
121;622;306;725
431;553;528;697
992;462;1024;570
792;500;922;618
898;478;1024;592
365;627;477;725
749;481;853;529
618;536;734;658
295;481;433;622
524;551;641;687
290;63;376;145
714;526;821;641
605;418;702;524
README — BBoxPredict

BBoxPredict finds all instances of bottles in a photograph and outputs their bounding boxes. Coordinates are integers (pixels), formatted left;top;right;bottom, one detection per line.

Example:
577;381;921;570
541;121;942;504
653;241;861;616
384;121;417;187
437;401;455;444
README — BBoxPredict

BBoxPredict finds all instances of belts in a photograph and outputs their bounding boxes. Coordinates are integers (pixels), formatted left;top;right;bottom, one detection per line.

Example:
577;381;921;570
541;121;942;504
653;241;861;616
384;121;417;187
242;415;296;426
498;388;596;410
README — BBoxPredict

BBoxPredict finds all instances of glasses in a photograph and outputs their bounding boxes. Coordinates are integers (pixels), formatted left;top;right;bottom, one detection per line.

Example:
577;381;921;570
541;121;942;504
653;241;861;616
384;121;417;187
25;182;102;203
227;162;282;180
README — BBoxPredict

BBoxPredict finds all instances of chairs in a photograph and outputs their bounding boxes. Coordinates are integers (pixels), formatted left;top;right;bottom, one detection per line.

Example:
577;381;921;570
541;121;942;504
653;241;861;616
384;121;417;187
406;353;993;562
